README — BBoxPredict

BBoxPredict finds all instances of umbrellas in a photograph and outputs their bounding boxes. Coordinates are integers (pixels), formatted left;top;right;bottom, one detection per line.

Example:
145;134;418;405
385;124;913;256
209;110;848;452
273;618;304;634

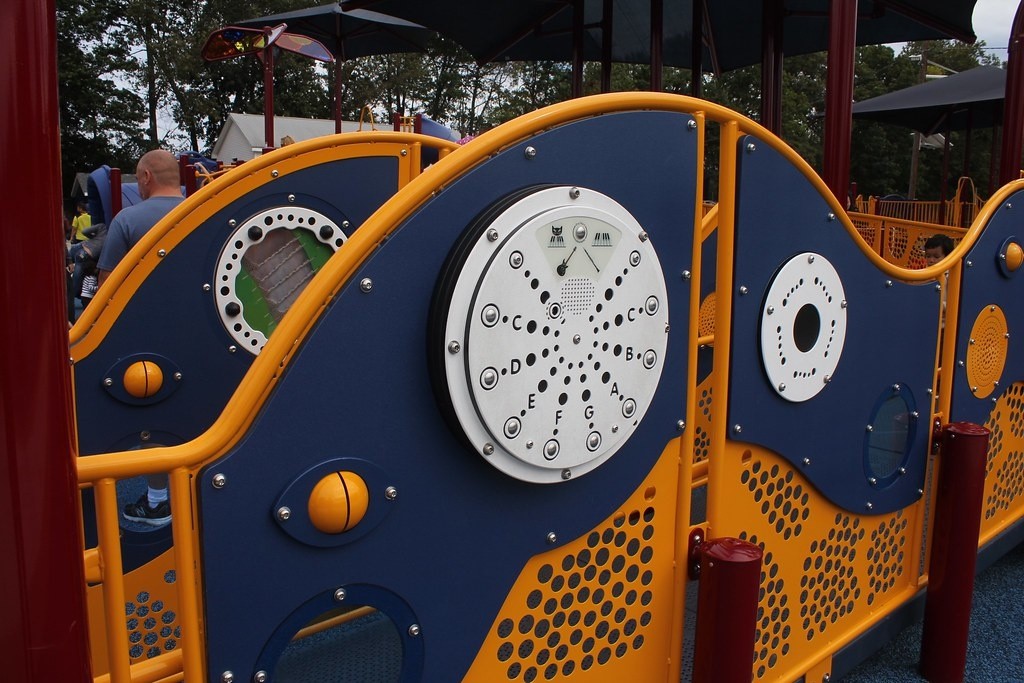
233;3;432;134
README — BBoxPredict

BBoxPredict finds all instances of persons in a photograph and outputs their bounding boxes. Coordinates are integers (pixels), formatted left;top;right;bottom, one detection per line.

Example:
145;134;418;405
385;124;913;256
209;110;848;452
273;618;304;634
925;234;955;392
97;149;187;525
65;242;75;329
64;215;71;241
72;223;107;298
69;205;91;244
81;263;98;326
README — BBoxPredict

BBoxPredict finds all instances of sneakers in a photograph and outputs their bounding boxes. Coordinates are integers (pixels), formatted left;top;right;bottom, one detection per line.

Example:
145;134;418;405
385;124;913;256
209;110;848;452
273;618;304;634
122;495;172;526
